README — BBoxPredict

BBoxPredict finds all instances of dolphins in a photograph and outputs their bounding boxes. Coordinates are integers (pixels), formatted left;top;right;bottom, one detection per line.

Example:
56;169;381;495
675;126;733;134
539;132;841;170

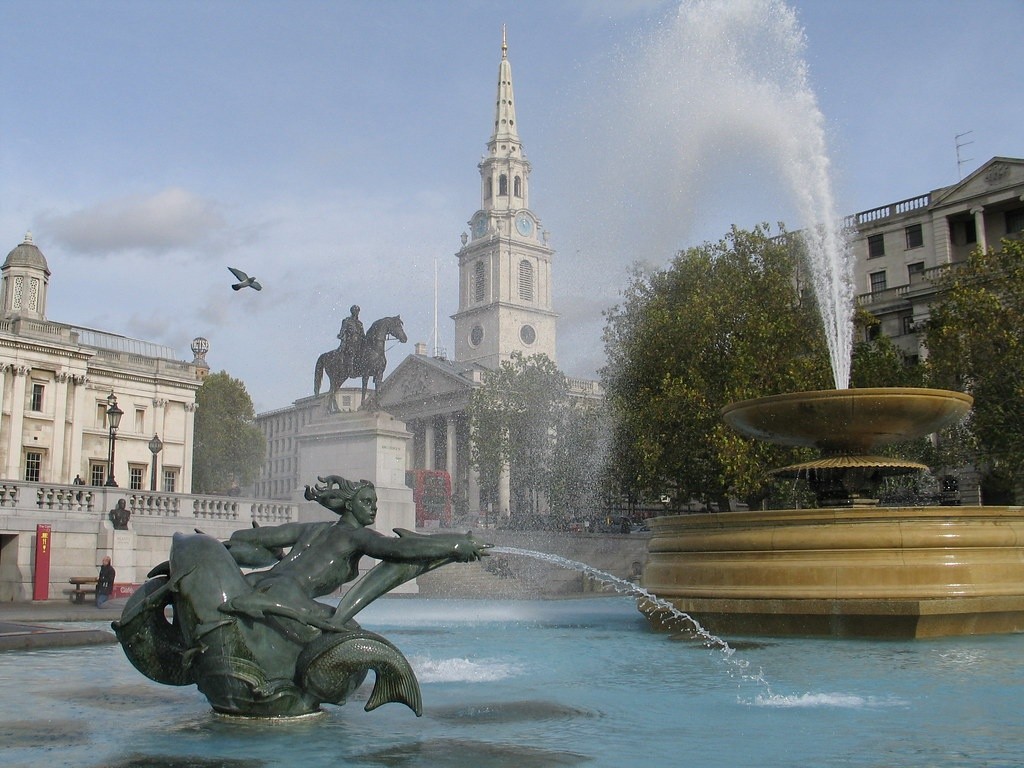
113;518;496;717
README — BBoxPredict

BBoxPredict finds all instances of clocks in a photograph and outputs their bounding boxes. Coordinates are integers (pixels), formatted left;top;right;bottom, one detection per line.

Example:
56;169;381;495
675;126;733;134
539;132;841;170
472;214;488;239
515;211;534;238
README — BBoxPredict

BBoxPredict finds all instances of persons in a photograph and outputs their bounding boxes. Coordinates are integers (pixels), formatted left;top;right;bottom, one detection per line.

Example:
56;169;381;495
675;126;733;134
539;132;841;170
95;555;115;607
337;305;365;380
110;499;130;530
74;474;83;500
227;473;481;706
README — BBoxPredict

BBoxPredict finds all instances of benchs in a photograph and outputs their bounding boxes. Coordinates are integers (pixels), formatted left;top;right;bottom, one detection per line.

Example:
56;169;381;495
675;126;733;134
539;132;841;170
62;576;100;606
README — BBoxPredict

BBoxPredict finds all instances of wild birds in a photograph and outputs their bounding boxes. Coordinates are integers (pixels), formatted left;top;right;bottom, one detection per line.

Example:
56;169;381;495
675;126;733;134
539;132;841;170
226;265;262;292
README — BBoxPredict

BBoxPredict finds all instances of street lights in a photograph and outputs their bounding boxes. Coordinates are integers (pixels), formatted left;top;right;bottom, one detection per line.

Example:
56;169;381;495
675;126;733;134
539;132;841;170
102;401;125;488
147;434;162;492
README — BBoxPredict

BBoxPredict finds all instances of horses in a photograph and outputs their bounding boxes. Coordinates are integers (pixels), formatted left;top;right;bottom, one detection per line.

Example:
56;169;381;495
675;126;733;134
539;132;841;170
313;313;408;416
